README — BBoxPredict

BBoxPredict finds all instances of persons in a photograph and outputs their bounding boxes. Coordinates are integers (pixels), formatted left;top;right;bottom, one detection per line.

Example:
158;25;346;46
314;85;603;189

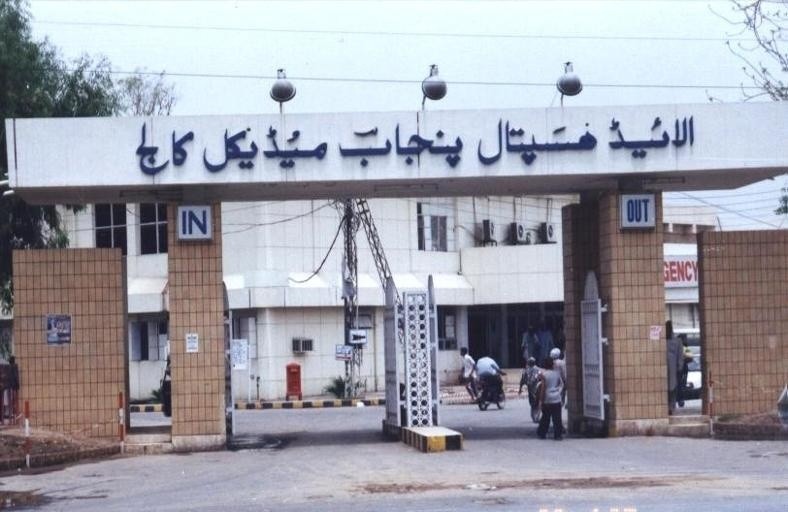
550;347;567;410
475;349;508;403
676;332;695;407
518;356;541;423
666;320;686;416
535;357;564;441
521;323;539;366
534;320;555;368
459;346;478;402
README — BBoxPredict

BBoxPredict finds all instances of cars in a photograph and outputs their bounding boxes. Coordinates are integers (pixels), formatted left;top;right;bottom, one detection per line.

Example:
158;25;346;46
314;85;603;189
685;354;702;399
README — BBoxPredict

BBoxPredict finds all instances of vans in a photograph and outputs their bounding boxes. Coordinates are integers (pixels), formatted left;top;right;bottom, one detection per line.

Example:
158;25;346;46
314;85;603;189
672;328;701;355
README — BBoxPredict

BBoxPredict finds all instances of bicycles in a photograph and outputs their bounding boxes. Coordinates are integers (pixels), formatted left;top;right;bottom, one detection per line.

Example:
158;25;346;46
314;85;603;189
477;374;506;411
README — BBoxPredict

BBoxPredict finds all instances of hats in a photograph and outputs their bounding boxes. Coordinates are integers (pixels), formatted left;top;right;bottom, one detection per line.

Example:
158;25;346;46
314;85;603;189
550;348;560;358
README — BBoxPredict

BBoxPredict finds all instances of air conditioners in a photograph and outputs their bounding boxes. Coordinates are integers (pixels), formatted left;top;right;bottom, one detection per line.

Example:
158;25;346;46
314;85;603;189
482;219;500;241
541;222;557;242
511;222;527;241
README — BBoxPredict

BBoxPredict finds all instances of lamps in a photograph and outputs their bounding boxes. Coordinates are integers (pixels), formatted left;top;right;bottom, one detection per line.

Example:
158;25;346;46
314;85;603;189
556;61;582;105
422;65;446;109
270;69;297;114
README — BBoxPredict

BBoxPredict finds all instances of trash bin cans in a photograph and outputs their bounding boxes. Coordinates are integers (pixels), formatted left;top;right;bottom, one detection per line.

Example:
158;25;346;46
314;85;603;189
286;364;304;401
